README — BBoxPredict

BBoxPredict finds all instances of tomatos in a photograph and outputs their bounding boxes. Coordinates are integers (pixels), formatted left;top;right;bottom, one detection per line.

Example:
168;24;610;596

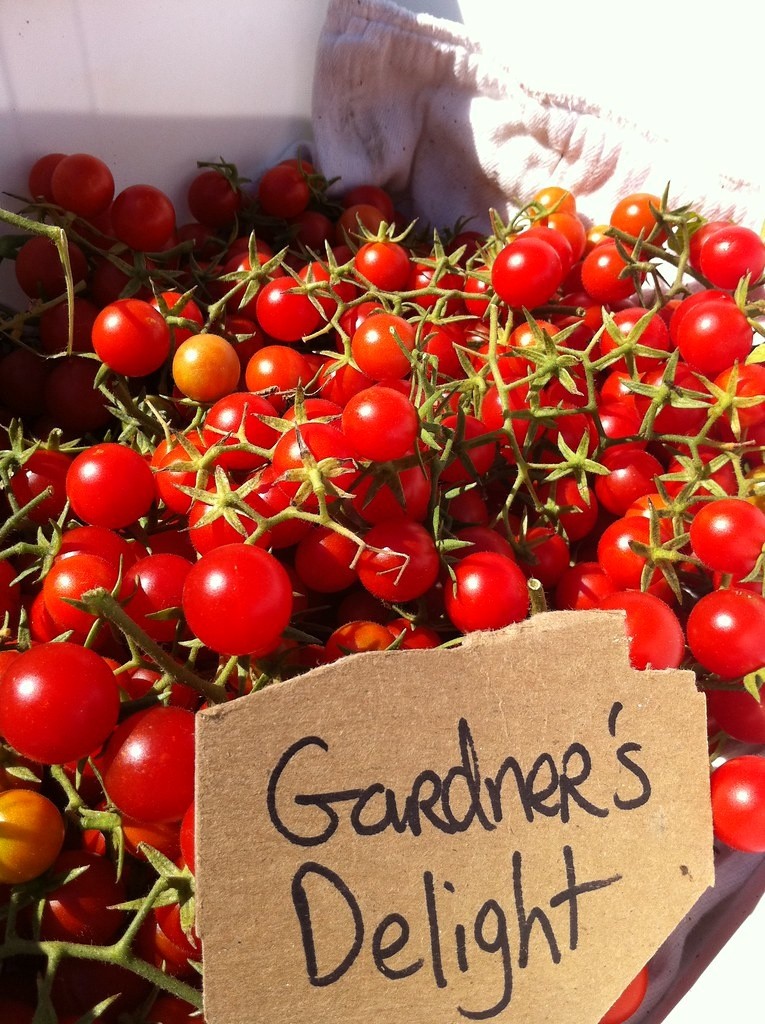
0;149;765;1022
